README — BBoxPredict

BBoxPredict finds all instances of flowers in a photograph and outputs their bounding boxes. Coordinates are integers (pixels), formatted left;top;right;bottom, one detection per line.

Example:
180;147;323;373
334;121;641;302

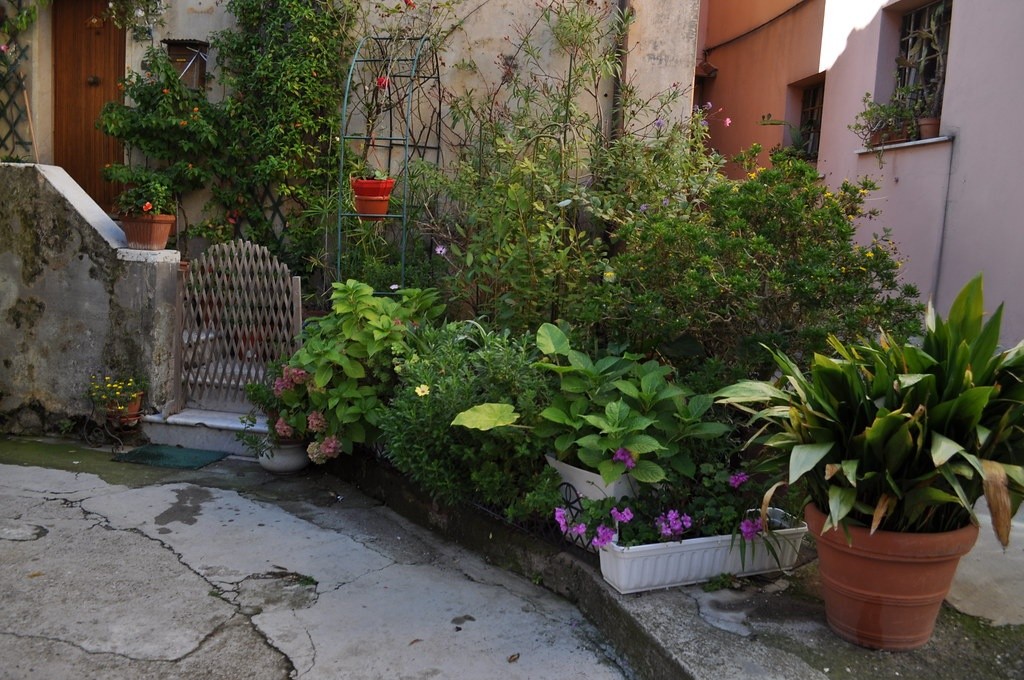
80;372;140;426
552;446;773;552
94;48;218;218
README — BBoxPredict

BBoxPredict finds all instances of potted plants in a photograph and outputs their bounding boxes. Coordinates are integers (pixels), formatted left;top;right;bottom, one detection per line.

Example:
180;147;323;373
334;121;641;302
448;322;733;554
232;341;311;477
711;272;1024;653
846;69;940;170
346;158;396;221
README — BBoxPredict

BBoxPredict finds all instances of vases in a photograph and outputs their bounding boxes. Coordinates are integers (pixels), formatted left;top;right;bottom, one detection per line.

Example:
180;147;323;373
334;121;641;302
594;507;809;596
104;392;144;426
122;211;176;250
